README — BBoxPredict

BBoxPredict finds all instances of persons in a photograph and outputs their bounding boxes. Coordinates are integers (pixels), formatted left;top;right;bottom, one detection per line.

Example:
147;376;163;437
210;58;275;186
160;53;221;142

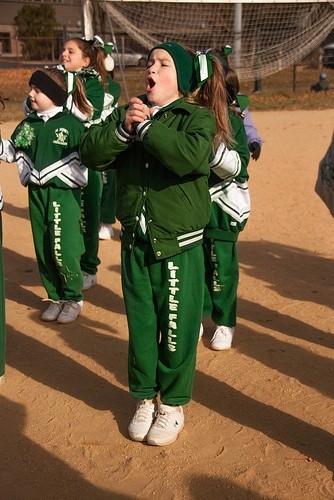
0;32;262;377
79;42;239;446
310;73;329;92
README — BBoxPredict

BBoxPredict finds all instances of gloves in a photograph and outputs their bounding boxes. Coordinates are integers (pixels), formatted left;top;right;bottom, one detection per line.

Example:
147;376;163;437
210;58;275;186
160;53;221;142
250;141;262;161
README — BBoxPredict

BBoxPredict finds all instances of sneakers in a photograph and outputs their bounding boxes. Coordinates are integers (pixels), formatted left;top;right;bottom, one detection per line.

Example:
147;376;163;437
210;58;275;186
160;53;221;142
127;399;158;443
145;403;185;447
40;298;65;322
98;225;114;240
209;325;236;352
80;270;98;290
57;300;84;324
198;323;204;340
